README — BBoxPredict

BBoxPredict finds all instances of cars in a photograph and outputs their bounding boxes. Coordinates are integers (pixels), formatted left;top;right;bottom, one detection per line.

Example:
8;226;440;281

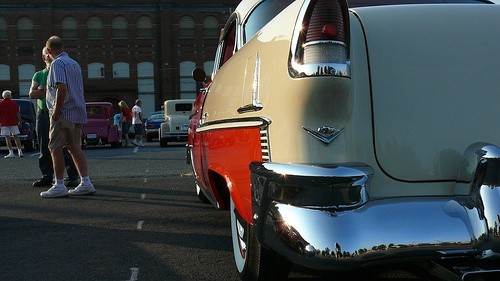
0;98;40;153
158;99;196;148
185;0;500;281
81;102;123;148
143;112;166;142
128;125;136;139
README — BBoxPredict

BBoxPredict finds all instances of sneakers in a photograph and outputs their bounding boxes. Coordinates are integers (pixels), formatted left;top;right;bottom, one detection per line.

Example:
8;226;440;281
68;183;95;195
40;183;68;197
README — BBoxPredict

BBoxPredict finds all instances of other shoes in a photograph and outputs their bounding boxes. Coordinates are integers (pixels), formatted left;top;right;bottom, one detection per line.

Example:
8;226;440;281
132;141;138;146
64;178;80;186
33;176;52;186
4;154;15;158
18;151;23;157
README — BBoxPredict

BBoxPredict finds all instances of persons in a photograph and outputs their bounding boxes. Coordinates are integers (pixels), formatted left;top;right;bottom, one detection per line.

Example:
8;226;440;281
29;47;79;188
131;99;144;147
41;36;96;196
118;101;131;147
0;90;24;159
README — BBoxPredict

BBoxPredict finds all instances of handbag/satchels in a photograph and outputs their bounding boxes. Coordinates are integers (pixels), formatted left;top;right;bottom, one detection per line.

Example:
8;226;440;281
120;116;127;122
